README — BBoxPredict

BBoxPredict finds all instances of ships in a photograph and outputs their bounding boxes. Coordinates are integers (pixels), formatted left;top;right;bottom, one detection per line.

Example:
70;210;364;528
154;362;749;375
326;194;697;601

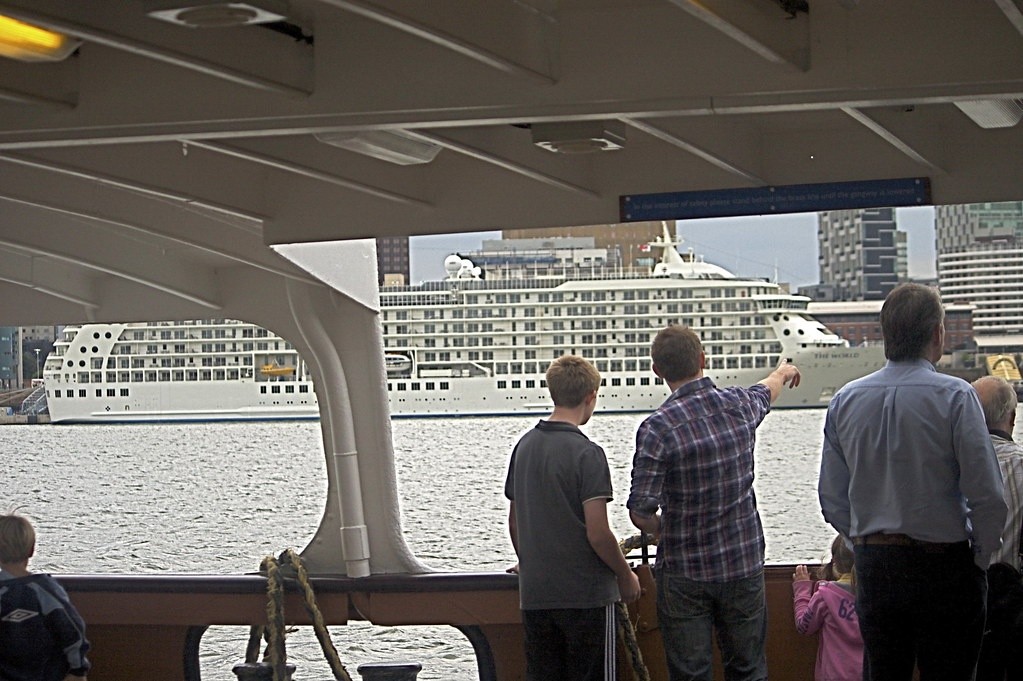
40;218;887;422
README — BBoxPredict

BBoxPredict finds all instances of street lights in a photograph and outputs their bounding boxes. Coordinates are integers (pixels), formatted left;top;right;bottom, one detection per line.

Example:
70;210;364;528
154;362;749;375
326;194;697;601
34;349;41;378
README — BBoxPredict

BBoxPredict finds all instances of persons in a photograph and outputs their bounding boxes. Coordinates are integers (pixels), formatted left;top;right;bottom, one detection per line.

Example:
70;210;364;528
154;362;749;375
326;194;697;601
0;514;91;681
625;326;801;681
819;282;1009;681
504;353;641;681
971;375;1023;680
791;533;864;681
